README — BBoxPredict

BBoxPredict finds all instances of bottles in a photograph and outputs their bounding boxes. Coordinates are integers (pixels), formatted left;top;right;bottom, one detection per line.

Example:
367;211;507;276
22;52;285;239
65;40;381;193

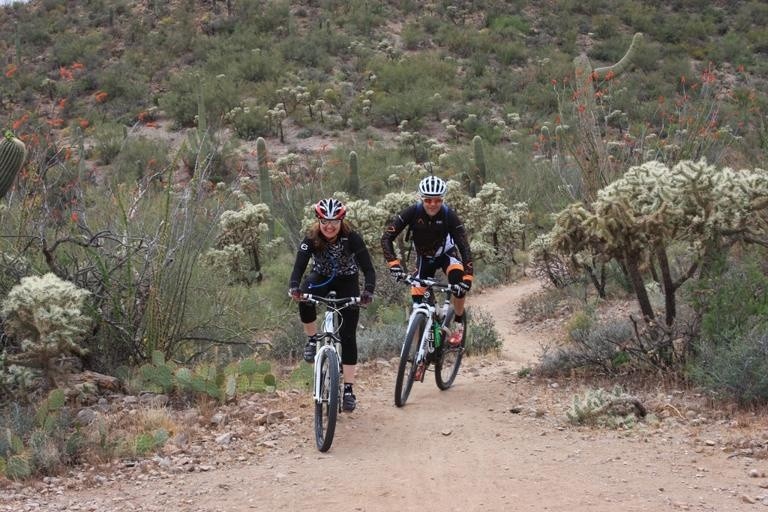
428;318;441;354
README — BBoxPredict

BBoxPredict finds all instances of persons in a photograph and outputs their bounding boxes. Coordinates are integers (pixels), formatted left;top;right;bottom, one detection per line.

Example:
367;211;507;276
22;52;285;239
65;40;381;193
381;176;473;347
288;198;376;412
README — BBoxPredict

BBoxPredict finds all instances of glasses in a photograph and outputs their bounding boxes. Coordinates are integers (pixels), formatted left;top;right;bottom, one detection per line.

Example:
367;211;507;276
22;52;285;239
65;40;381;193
319;220;341;225
422;197;443;204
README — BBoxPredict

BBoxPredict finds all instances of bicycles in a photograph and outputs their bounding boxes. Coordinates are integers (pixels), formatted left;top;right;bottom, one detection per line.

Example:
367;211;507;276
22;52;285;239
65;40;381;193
390;270;467;407
290;291;374;452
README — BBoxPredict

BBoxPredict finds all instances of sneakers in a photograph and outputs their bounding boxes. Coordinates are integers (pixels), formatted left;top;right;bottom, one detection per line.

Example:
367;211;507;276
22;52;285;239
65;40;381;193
412;361;425;381
343;393;356;411
304;334;316;362
448;320;465;347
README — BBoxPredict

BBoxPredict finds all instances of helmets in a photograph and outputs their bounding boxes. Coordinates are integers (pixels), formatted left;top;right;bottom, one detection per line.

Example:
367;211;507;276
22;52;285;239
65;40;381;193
419;175;446;197
314;198;346;219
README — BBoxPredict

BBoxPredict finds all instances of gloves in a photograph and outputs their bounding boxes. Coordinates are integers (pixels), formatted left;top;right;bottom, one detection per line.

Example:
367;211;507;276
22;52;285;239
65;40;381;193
362;294;372;304
387;264;403;278
454;280;472;295
291;288;303;300
